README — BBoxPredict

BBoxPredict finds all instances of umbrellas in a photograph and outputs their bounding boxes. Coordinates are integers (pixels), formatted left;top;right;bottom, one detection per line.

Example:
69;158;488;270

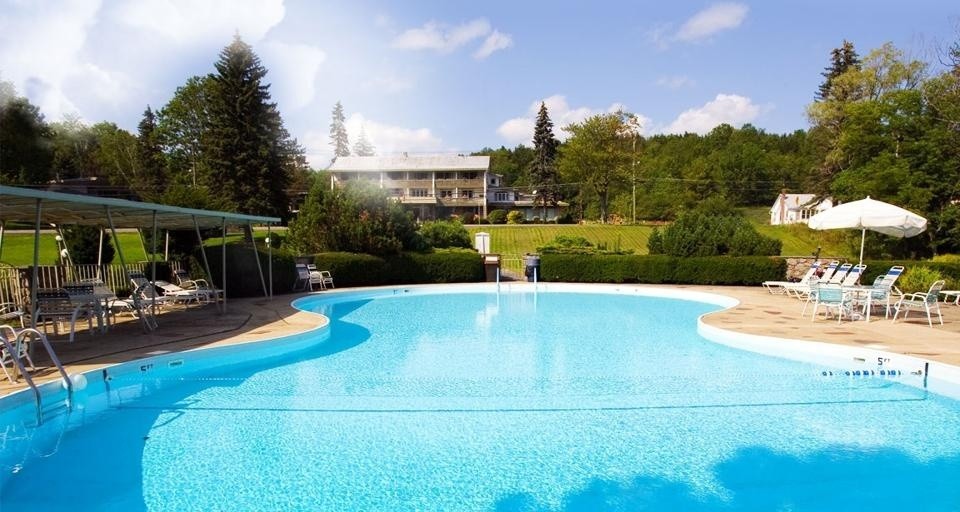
806;194;928;311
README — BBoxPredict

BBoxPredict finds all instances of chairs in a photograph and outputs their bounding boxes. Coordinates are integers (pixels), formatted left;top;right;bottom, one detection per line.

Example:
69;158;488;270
0;270;225;385
292;263;334;292
762;261;960;326
